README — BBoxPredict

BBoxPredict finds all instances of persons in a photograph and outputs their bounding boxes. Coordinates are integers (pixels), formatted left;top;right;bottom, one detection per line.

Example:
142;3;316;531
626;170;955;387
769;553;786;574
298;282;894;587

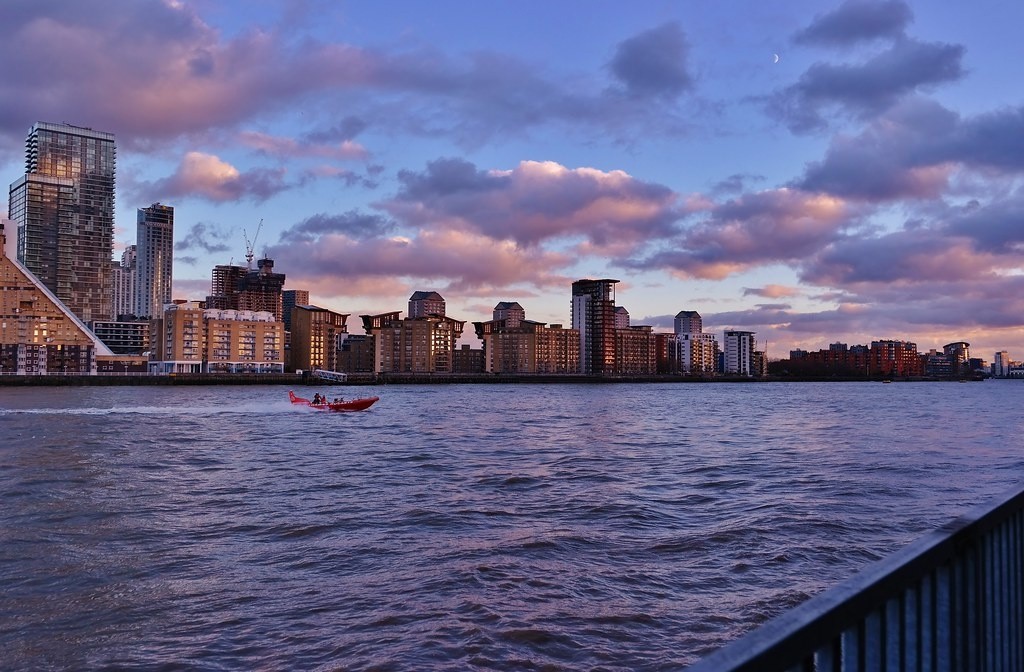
312;392;327;404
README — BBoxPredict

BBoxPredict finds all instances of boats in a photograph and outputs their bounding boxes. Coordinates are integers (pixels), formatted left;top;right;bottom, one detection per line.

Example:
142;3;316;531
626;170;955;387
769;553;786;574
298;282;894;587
883;380;891;383
288;390;379;411
959;380;967;383
